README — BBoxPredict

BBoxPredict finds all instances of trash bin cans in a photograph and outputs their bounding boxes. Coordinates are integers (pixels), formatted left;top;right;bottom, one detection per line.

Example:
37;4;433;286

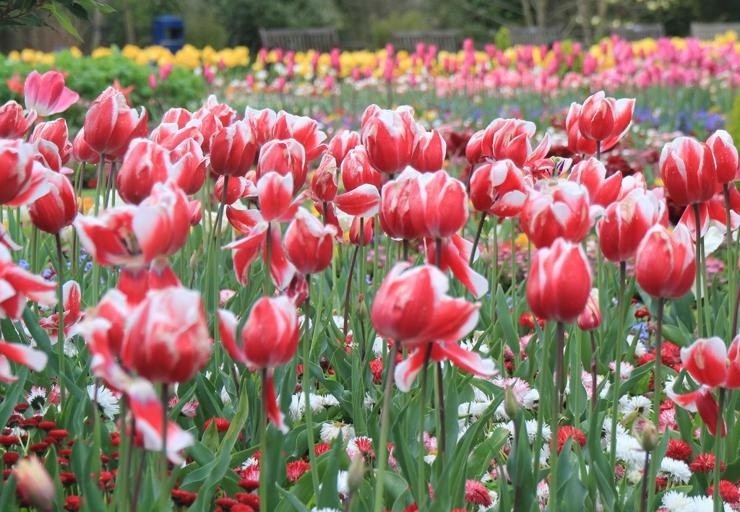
152;16;184;54
